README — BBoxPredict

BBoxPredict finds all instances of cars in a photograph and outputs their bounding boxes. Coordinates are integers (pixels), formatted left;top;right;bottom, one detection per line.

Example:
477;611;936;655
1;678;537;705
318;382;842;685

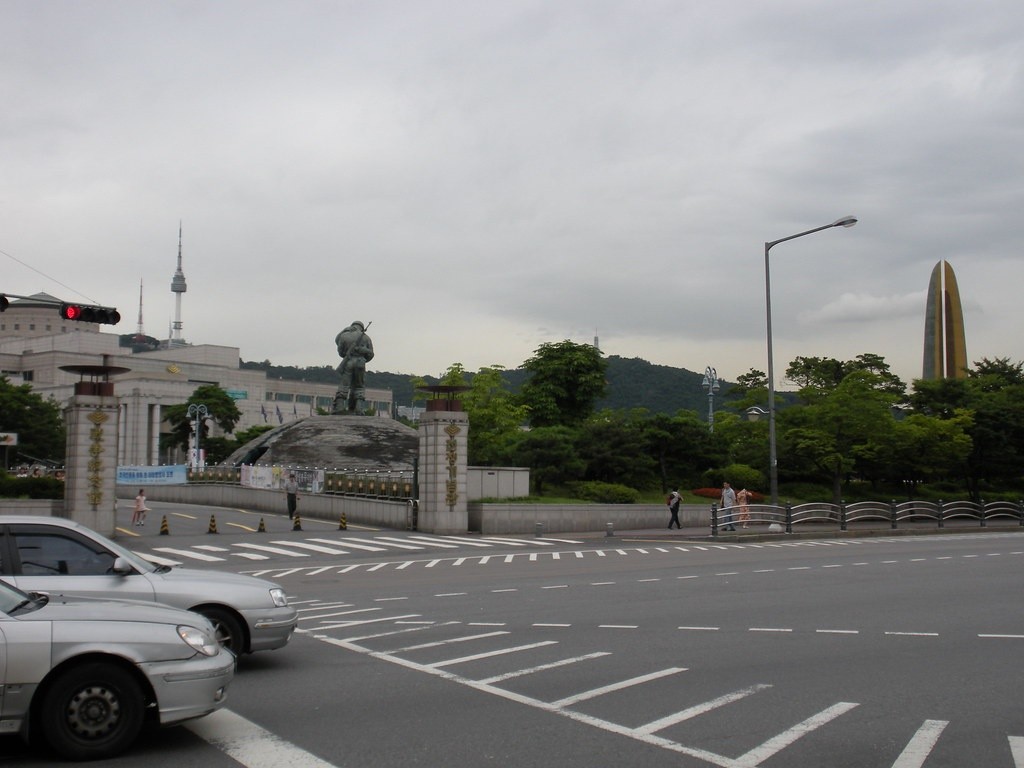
0;514;300;666
0;577;237;759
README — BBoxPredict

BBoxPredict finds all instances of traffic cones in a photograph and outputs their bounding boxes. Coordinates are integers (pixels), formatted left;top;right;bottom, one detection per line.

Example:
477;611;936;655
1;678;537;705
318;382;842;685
257;517;266;533
292;515;303;531
338;512;347;530
159;514;170;536
206;514;219;534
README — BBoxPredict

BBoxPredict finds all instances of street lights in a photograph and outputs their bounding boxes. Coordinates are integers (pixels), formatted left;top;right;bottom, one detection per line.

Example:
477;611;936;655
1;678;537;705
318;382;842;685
702;366;720;433
745;214;859;522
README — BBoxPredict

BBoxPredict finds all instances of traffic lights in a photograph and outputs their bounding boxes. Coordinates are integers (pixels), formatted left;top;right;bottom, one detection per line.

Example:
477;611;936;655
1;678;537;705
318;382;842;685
59;303;121;326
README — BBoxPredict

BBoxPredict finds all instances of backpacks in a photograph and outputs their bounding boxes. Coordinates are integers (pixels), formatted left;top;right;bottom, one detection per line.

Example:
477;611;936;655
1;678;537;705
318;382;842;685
666;492;676;506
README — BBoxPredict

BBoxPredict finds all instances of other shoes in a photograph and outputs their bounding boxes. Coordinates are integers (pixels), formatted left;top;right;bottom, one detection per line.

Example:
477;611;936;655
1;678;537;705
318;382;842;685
140;521;144;525
728;528;736;531
135;522;140;526
721;527;727;531
669;527;673;530
678;526;682;529
742;525;749;529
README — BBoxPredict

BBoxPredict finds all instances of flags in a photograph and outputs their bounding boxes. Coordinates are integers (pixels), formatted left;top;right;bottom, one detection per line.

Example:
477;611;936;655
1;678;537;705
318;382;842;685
260;405;267;422
275;405;283;424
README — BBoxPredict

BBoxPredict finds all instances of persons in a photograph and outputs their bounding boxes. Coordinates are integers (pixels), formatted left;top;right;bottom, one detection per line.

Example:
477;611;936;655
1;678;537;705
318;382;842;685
285;474;300;520
719;481;738;531
737;486;753;528
333;320;373;412
135;488;152;525
15;467;67;481
334;326;374;412
667;486;684;529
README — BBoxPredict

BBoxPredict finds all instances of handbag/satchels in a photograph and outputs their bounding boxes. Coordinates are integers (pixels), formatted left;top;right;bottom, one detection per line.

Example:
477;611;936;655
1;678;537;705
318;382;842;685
721;496;724;511
745;490;751;504
